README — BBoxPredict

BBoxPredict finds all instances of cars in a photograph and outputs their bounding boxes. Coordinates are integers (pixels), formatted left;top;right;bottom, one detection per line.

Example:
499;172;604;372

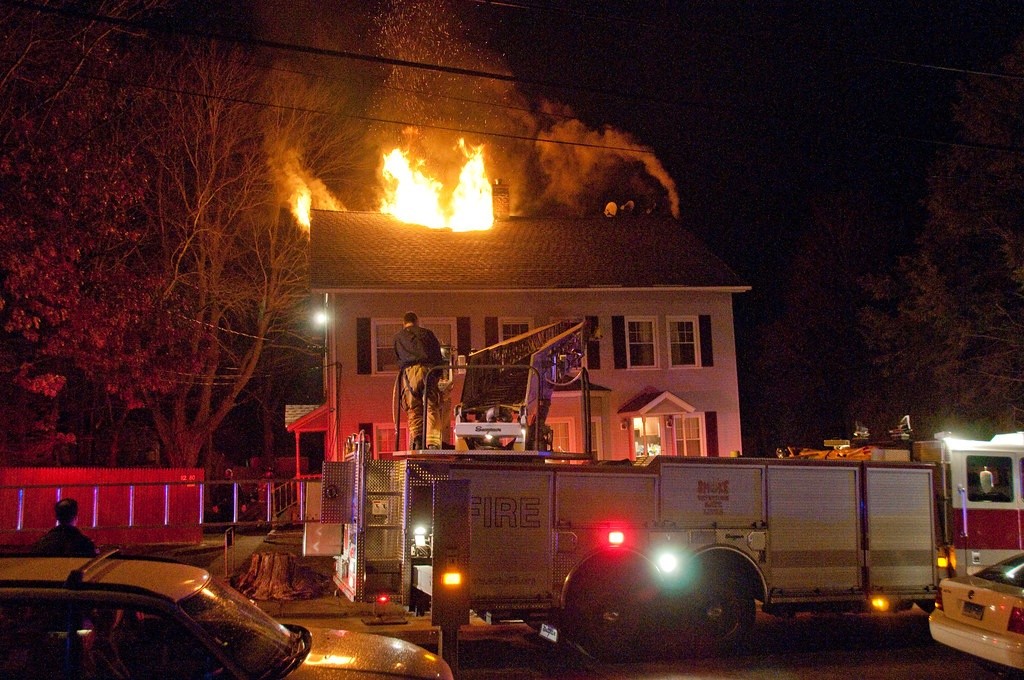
929;554;1024;679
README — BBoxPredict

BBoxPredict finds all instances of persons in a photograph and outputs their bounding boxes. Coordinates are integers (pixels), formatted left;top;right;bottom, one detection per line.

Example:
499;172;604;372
24;497;100;558
212;468;247;522
250;466;283;518
394;311;444;451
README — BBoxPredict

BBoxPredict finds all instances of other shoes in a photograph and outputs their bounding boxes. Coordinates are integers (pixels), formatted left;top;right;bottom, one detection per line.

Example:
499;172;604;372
428;445;439;451
413;438;427;450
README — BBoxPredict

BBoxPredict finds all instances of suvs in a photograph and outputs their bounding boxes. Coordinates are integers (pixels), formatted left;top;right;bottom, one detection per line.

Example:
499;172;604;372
0;544;453;680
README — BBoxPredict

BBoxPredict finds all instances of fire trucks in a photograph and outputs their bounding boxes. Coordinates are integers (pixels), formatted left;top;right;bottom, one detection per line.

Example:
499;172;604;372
322;319;1024;663
